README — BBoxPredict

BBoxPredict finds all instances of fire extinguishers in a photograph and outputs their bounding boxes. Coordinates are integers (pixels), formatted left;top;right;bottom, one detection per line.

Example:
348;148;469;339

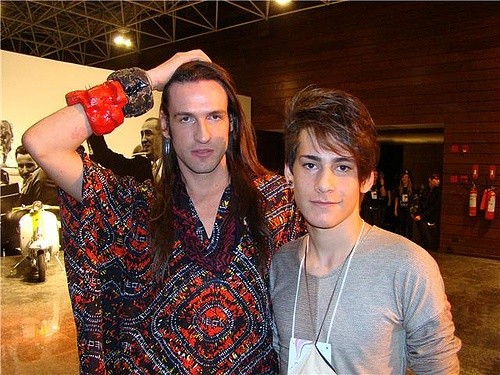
469;183;478;216
485;186;497;220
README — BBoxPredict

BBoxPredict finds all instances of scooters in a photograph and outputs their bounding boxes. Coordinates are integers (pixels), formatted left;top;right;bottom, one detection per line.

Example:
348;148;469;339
10;200;65;282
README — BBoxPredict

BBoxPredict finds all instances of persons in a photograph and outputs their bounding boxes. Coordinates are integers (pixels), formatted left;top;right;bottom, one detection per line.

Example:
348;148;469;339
363;169;442;251
0;116;164;218
22;49;309;375
270;85;462;374
